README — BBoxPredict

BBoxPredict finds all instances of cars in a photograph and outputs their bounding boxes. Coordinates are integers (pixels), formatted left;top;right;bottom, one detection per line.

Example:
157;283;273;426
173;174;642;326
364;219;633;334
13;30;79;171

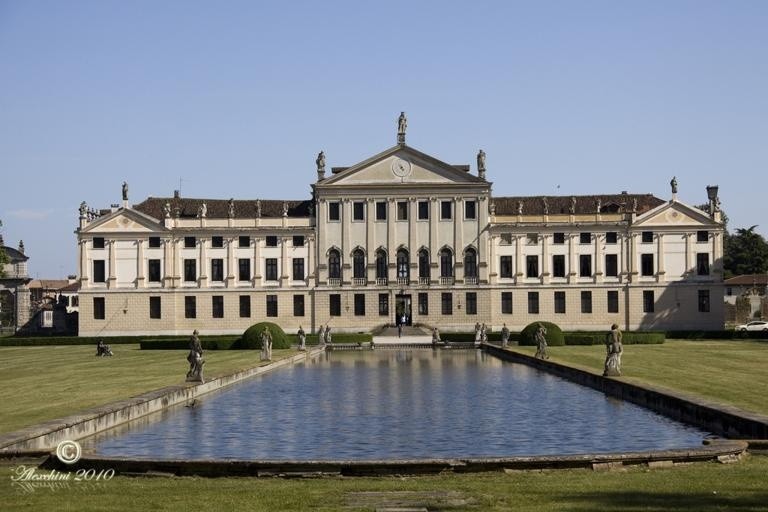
735;321;768;331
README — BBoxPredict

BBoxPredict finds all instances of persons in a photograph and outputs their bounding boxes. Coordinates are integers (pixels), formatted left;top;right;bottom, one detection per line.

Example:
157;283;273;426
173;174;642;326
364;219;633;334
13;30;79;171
601;325;624;376
317;324;325;344
396;310;412;326
297;326;305;350
317;150;327;170
500;323;510;347
398;110;407;134
122;180;128;199
399;324;404;338
474;322;482;343
432;328;441;343
259;327;272;363
97;338;113;357
326;325;332;344
671;176;679;193
535;322;549;358
481;323;488;343
187;328;204;383
477;149;486;171
